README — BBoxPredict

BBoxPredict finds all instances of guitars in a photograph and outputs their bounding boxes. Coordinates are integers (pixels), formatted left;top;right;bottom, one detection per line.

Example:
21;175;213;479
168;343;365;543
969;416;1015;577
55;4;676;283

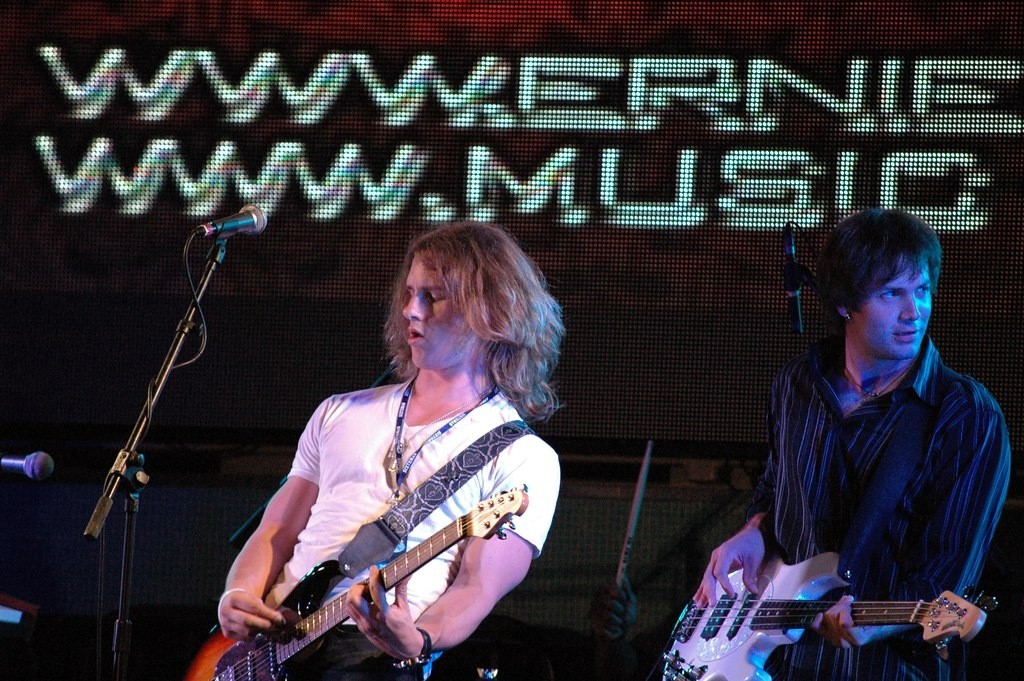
183;482;530;681
659;549;1002;681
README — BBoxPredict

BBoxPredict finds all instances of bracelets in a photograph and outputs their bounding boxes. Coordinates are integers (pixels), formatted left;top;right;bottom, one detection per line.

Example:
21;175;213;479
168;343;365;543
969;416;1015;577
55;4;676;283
218;588;245;623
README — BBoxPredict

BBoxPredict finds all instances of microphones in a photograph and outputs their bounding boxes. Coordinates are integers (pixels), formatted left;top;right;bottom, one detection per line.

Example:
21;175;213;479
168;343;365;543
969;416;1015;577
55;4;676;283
784;224;804;334
0;451;55;482
194;205;268;238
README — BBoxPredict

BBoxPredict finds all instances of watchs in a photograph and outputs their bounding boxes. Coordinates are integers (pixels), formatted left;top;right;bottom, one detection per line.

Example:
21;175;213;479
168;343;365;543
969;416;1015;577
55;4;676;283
392;627;432;670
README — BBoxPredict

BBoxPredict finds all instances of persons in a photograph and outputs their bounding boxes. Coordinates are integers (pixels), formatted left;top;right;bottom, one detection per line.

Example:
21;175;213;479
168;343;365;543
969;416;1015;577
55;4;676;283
217;221;565;681
591;575;638;681
692;208;1011;680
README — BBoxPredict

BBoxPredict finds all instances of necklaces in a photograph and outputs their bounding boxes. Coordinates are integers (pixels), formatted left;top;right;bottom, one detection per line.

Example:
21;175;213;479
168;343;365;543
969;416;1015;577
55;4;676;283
389;383;497;471
845;360;914;398
